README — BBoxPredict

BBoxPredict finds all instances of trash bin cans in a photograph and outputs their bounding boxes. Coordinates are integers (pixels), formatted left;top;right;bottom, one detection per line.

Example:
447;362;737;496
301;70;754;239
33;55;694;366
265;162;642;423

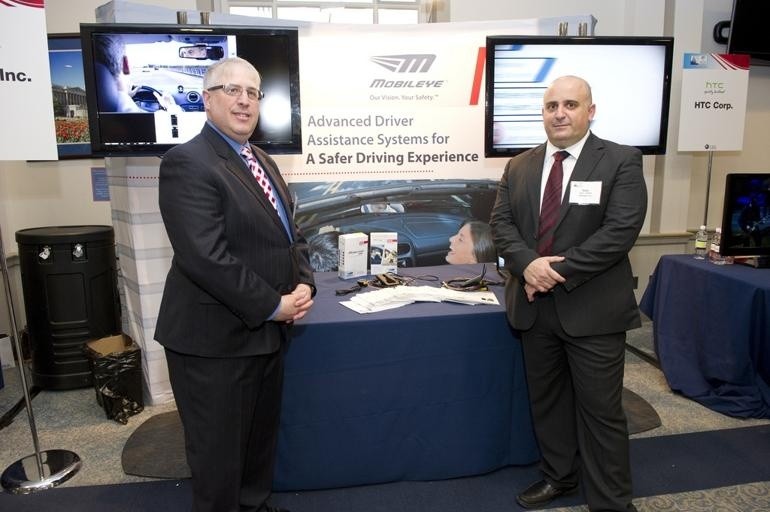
83;332;144;425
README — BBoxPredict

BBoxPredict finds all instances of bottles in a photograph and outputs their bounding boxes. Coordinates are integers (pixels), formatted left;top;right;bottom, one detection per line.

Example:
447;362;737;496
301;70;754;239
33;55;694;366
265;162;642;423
696;226;707;259
709;228;719;263
714;228;725;265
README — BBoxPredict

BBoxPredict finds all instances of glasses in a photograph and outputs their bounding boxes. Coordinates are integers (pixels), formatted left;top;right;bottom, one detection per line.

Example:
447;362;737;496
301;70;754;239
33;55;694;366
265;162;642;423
207;83;264;100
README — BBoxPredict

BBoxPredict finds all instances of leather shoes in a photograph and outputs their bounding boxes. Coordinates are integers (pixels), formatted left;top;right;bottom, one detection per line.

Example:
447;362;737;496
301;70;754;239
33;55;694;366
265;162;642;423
516;480;575;509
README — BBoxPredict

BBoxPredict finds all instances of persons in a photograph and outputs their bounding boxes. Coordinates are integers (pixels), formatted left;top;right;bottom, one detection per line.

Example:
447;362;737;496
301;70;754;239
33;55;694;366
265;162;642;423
445;219;498;264
93;35;183;113
154;56;317;512
181;48;207;58
490;75;648;512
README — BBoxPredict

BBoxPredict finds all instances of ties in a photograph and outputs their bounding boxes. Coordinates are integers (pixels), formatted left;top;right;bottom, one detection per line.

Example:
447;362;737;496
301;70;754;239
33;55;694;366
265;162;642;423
537;149;570;255
241;147;278;212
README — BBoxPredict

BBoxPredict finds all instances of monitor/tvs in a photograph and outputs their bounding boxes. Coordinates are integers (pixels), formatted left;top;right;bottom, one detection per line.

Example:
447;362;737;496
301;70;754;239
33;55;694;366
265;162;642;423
719;172;770;257
726;0;770;67
482;35;676;159
79;22;304;159
25;32;104;163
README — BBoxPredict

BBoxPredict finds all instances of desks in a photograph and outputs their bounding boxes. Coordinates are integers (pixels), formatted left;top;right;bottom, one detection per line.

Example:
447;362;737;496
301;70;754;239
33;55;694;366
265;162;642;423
257;262;556;493
650;252;769;385
